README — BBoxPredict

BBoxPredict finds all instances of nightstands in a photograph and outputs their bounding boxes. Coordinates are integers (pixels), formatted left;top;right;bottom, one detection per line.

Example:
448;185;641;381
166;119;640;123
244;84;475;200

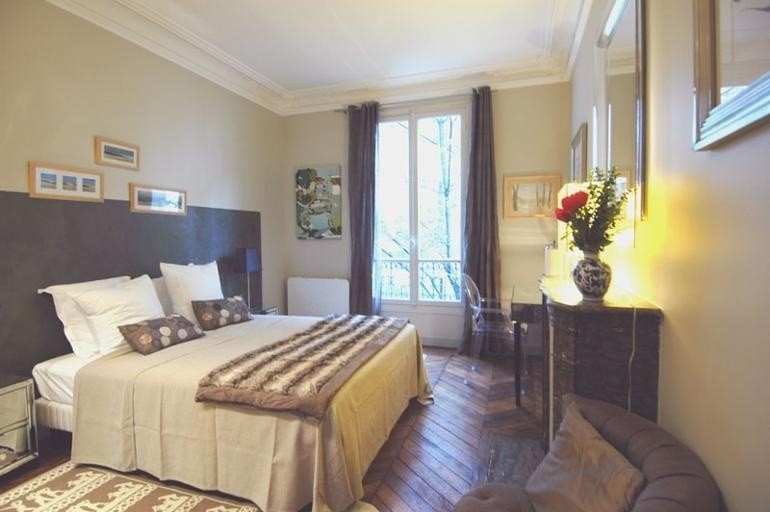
260;302;280;316
1;371;44;479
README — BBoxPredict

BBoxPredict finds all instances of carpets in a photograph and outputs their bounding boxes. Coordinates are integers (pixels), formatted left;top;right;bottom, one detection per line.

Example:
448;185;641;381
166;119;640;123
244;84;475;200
0;459;382;511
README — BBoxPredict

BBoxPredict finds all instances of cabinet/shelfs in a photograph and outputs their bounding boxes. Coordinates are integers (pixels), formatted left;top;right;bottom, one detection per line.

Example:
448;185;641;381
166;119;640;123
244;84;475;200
539;283;664;455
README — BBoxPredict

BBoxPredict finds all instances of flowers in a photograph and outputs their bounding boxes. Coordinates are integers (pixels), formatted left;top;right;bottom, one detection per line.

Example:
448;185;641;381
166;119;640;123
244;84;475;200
546;165;638;255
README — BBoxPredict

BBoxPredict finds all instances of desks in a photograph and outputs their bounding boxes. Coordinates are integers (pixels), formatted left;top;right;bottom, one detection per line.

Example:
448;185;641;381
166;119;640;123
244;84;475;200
506;281;548;411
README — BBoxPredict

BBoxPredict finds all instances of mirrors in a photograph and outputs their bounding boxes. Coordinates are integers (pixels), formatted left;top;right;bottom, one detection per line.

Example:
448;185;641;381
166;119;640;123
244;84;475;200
598;2;650;224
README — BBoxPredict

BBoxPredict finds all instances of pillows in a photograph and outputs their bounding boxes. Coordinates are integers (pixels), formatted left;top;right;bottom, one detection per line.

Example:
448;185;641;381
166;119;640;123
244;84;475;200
148;272;178;316
37;273;131;354
156;258;223;325
191;294;256;331
117;314;205;354
73;274;167;352
520;403;649;510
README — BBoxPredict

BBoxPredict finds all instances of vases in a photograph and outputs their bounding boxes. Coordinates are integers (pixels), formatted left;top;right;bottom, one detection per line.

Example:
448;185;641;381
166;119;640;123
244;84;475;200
573;248;614;304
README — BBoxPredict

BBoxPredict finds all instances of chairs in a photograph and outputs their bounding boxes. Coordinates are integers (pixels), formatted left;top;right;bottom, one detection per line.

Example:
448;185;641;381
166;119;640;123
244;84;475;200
454;271;535;386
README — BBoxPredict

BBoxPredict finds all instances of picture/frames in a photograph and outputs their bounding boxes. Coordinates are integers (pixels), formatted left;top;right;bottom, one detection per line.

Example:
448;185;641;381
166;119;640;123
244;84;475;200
126;182;187;217
25;160;105;204
570;123;588;184
93;136;143;172
502;175;562;221
691;0;770;150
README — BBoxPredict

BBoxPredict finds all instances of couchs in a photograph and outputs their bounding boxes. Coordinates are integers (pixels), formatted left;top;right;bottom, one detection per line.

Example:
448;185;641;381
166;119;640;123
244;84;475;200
451;390;739;511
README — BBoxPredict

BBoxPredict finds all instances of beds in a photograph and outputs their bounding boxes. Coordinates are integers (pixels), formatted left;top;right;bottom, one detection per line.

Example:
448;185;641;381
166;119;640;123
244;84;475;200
29;253;438;511
